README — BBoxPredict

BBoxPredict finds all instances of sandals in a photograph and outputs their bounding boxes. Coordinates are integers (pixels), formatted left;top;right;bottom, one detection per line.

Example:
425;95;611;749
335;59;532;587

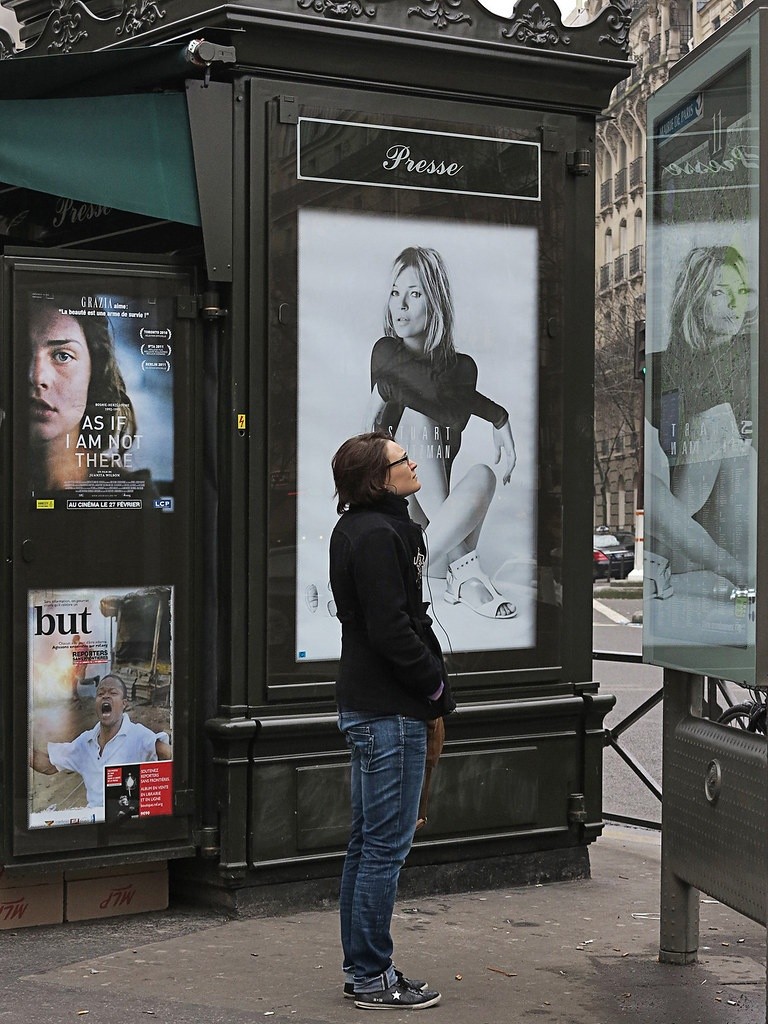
643;550;674;603
444;550;518;619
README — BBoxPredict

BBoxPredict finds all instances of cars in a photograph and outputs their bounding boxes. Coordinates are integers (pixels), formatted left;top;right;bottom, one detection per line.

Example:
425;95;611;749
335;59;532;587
593;525;636;579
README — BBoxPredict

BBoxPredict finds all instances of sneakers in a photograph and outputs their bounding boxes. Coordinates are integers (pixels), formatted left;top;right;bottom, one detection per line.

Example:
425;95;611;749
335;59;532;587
343;974;428;996
354;983;442;1011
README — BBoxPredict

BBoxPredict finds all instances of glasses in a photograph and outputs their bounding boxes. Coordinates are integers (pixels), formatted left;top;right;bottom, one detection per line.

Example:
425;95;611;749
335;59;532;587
384;454;410;469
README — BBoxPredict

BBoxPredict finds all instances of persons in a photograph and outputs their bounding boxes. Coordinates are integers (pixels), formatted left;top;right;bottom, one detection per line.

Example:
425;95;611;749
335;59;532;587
637;245;759;609
327;246;522;621
28;290;159;498
324;431;455;1011
27;673;171;812
